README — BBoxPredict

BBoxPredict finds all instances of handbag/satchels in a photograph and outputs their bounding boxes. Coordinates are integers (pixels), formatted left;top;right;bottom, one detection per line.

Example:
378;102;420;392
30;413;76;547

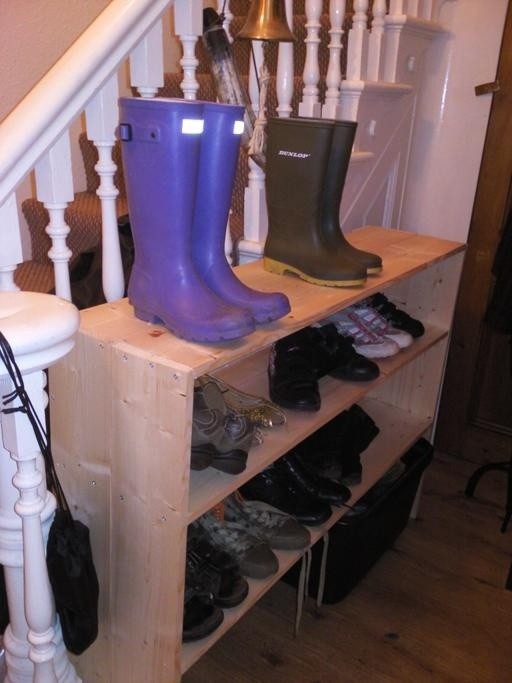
45;508;101;655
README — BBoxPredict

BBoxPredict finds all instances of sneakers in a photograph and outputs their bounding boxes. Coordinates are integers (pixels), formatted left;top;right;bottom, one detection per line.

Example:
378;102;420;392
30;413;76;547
365;292;426;341
198;511;282;581
294;402;380;485
324;309;400;364
212;487;312;552
266;330;323;412
190;407;254;476
309;324;383;383
351;302;416;349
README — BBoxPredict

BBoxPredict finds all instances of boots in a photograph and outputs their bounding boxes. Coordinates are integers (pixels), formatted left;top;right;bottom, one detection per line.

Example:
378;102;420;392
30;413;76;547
169;96;293;326
286;110;386;274
116;94;257;345
261;114;369;289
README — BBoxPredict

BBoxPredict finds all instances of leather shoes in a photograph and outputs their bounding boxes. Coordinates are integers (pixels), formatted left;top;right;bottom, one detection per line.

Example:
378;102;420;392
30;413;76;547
197;374;288;446
241;466;333;528
276;452;352;508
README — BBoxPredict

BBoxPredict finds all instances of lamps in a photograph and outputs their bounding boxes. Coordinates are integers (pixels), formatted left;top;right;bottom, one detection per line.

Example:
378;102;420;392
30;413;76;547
232;1;298;156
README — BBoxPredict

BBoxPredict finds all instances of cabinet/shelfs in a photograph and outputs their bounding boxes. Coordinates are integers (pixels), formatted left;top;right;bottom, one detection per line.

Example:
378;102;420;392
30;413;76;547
39;225;466;683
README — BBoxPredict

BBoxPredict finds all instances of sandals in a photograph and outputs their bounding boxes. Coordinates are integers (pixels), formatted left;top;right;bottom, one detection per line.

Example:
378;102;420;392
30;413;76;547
183;526;251;610
180;583;226;643
